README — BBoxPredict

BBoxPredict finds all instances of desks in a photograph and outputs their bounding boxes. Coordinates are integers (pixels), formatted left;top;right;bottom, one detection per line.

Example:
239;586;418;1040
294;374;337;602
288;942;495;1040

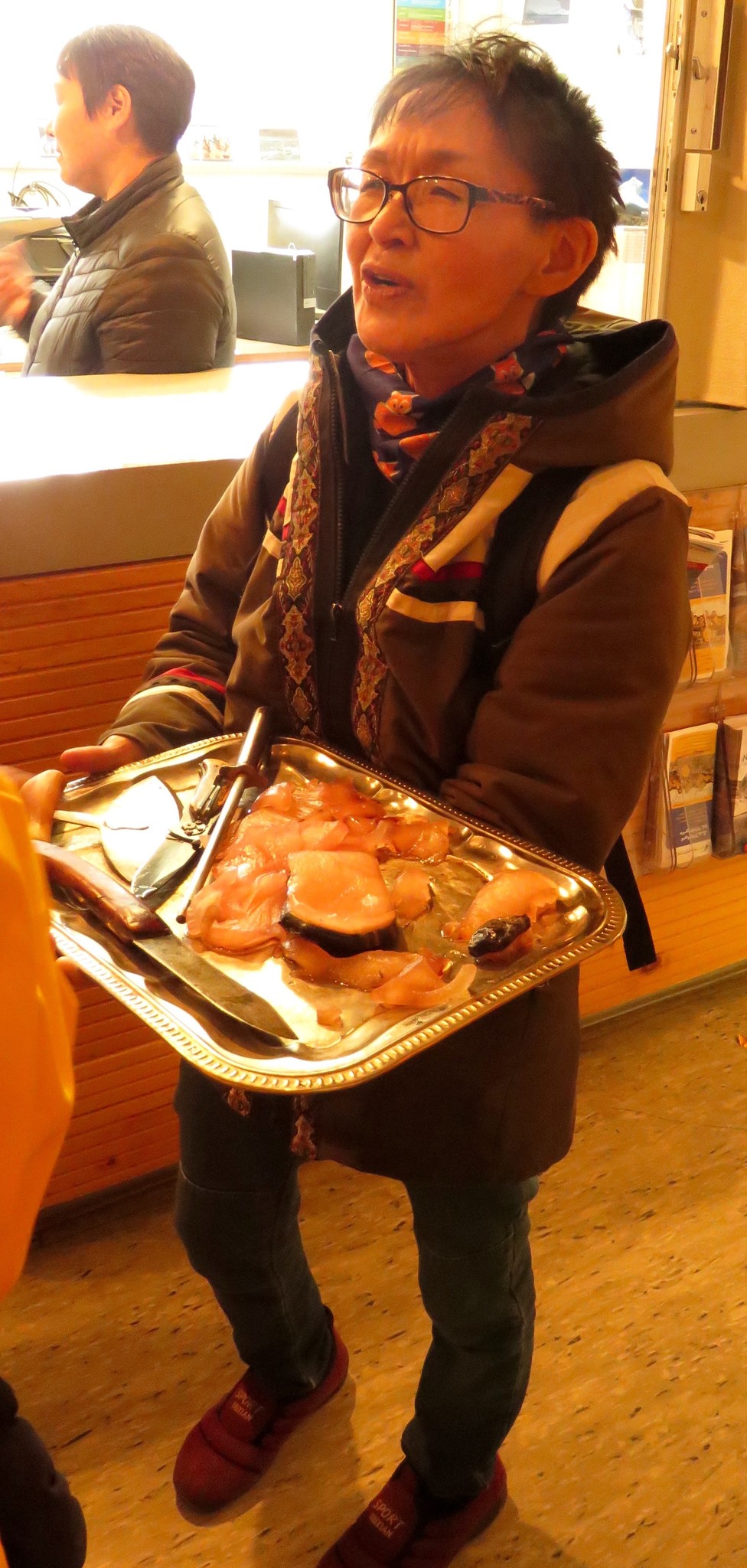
234;325;311;363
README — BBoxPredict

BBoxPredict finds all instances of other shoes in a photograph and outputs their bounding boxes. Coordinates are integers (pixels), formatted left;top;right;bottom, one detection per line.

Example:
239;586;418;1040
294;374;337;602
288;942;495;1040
172;1305;348;1512
313;1444;510;1568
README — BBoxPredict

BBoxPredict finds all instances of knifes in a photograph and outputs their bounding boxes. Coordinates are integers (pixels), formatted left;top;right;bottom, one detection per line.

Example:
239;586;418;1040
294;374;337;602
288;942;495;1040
30;839;300;1041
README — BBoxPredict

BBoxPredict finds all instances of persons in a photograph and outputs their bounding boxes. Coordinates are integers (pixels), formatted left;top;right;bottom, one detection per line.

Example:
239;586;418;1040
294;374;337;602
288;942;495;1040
0;26;234;378
58;26;696;1568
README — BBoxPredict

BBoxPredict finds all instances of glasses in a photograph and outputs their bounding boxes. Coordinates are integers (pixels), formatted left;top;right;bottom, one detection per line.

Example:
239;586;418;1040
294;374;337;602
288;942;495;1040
328;166;567;238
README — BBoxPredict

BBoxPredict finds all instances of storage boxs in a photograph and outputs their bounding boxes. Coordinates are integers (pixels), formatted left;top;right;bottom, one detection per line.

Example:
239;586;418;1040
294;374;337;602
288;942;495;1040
231;249;317;346
